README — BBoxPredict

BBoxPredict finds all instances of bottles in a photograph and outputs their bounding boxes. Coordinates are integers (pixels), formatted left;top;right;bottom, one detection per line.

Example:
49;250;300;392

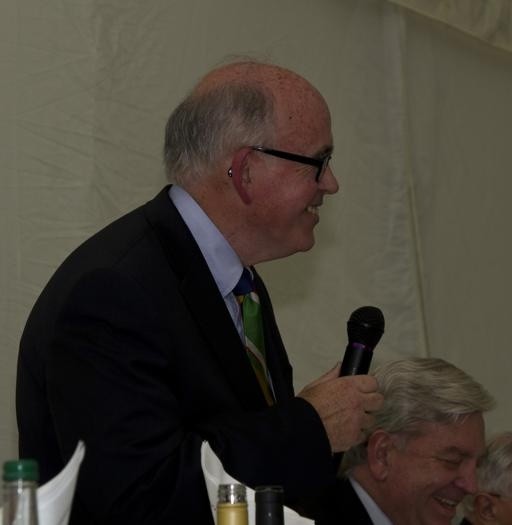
3;457;39;524
217;484;248;525
254;485;284;525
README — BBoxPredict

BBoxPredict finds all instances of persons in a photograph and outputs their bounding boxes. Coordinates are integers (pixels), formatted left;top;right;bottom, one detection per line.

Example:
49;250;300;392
14;60;385;525
328;356;497;525
451;431;512;525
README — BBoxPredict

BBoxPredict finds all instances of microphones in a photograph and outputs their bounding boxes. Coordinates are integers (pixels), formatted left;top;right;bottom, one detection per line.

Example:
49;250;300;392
339;305;384;377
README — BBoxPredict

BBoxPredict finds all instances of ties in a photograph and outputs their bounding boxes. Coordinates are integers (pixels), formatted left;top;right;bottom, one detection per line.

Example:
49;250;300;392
231;266;279;410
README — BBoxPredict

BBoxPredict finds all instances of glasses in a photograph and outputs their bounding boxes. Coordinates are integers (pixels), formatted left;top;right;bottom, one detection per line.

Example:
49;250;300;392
225;135;333;184
